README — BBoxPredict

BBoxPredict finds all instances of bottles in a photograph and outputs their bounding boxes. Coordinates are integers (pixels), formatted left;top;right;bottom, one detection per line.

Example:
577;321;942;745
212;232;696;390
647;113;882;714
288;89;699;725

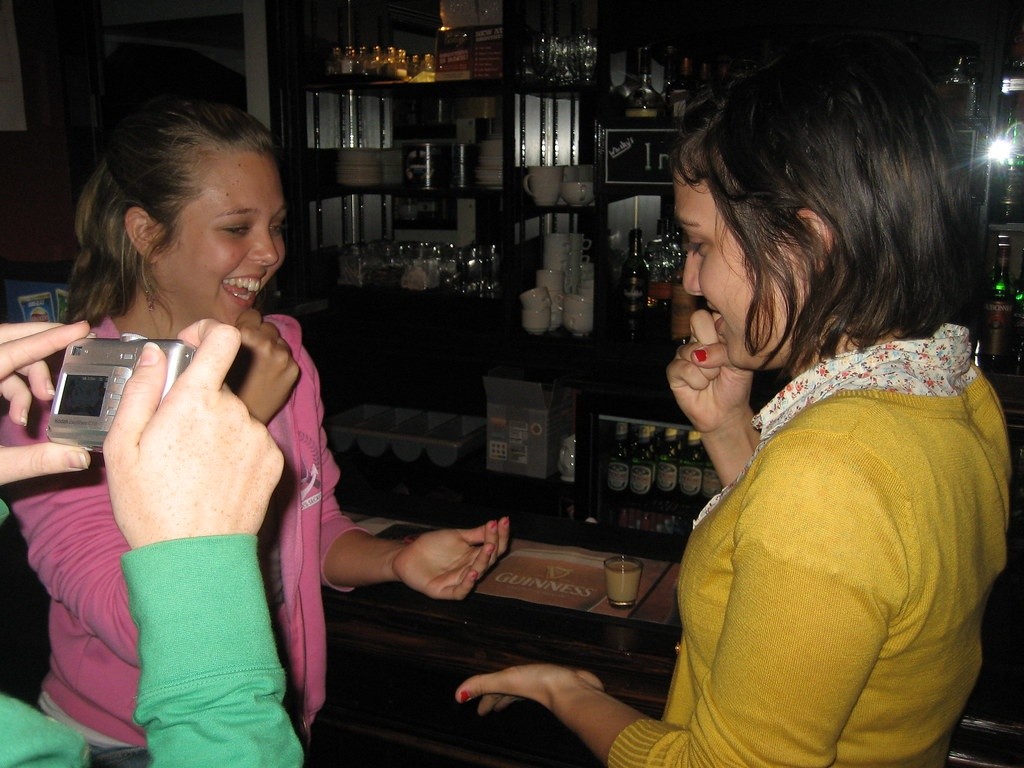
995;93;1024;223
619;229;650;340
608;421;722;507
324;46;434;81
979;234;1024;377
670;261;696;344
624;46;728;120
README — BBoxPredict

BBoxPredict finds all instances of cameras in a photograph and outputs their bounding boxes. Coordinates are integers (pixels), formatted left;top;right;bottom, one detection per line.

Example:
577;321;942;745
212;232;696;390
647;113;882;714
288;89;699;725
46;332;198;454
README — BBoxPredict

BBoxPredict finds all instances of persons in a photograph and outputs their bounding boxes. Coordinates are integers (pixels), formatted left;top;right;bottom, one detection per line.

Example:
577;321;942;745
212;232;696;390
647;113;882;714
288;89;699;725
453;31;1013;768
0;95;510;768
0;318;303;767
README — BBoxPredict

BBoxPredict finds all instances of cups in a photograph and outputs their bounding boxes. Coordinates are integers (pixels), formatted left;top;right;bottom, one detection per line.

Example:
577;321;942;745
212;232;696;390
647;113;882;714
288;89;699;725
558;434;574;481
339;239;498;291
532;27;596;82
603;555;643;608
561;166;594;206
524;165;563;206
520;232;594;337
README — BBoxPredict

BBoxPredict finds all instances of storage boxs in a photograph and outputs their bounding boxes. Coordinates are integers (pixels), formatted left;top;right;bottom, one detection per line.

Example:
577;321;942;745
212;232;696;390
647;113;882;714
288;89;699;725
481;365;576;479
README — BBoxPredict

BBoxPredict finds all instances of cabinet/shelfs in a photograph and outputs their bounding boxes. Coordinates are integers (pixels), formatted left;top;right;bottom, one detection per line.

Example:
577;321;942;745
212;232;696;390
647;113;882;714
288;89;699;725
267;1;1024;535
